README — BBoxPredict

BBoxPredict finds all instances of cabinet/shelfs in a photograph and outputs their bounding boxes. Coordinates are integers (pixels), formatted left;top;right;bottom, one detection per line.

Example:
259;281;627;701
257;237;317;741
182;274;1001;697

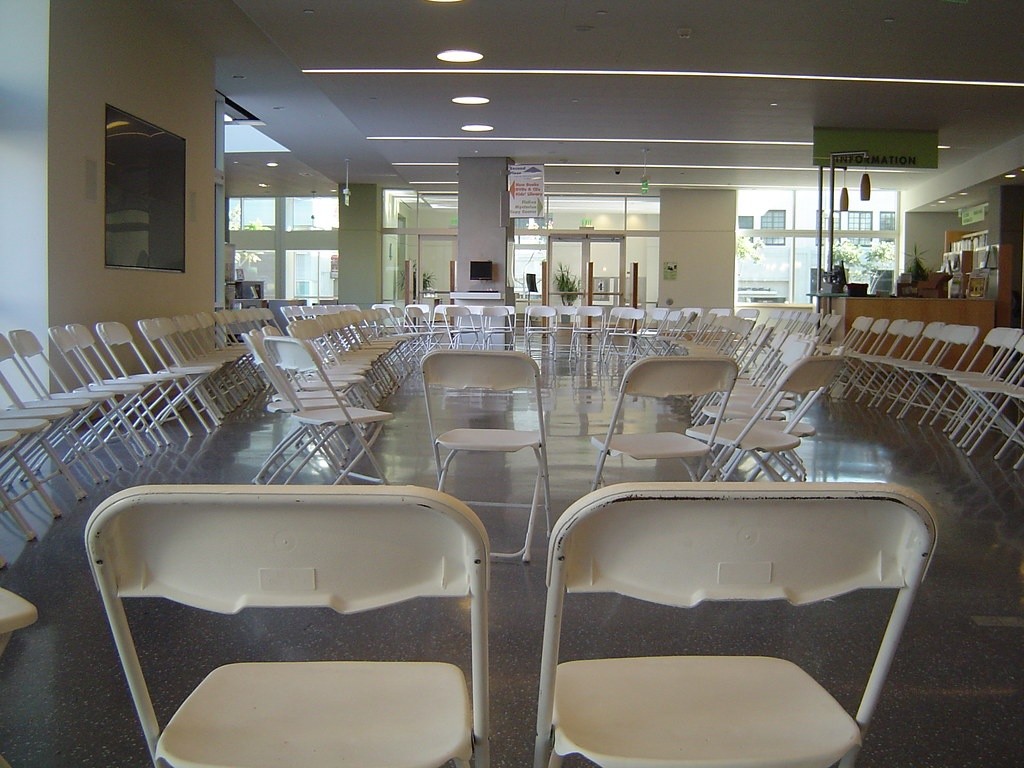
941;229;999;299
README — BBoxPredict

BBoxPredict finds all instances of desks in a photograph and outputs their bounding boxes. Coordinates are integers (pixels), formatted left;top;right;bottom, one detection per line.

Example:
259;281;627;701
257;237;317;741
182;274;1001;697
820;296;996;385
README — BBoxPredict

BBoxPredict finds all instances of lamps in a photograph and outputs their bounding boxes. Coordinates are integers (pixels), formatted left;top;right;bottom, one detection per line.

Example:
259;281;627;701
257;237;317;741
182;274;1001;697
840;168;849;212
861;172;871;202
343;160;352;207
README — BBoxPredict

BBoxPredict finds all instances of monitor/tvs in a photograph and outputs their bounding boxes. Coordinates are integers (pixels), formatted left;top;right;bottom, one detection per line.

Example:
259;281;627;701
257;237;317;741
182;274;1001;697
470;261;492;279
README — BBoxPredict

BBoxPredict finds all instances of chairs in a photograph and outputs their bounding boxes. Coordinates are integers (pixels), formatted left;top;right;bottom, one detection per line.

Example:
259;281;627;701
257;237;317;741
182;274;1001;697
84;483;491;768
534;482;940;768
0;305;1024;659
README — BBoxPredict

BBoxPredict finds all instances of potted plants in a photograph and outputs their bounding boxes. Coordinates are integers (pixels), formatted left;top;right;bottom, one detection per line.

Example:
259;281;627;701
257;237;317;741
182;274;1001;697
555;263;580;322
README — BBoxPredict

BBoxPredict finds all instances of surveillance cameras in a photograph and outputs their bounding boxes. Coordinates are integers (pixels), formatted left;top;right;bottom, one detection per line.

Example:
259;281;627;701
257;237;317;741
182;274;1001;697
614;168;621;175
